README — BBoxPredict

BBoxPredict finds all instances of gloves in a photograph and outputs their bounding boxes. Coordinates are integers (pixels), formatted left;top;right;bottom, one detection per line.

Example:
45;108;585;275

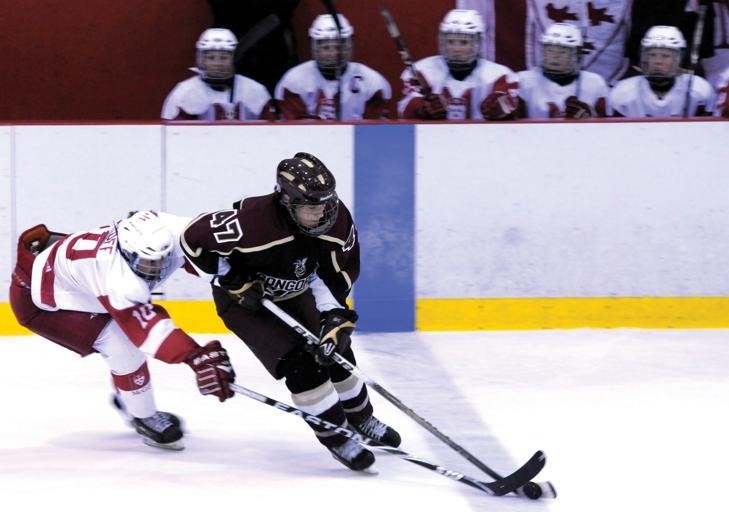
481;91;519;119
414;94;447;120
185;340;235;402
221;268;275;311
565;95;591;117
316;315;356;366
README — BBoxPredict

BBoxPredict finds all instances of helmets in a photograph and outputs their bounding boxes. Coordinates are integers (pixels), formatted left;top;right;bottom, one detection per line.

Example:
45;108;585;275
439;9;484;65
277;152;339;235
641;26;687;76
196;28;239;80
540;24;583;75
308;14;354;68
118;211;175;282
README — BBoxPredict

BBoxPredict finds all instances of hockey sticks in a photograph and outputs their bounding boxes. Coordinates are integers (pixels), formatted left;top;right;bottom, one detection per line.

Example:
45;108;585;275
319;0;343;119
262;297;558;500
227;383;545;495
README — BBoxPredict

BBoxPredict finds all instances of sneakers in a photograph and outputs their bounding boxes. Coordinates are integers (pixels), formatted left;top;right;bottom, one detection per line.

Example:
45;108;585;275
134;410;183;443
329;439;375;470
347;414;401;448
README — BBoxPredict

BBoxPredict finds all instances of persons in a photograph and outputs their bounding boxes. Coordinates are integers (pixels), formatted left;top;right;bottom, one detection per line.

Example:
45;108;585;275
159;27;273;121
608;26;729;120
482;19;606;120
8;210;233;443
397;8;512;117
686;1;728;75
274;13;391;120
179;154;402;471
